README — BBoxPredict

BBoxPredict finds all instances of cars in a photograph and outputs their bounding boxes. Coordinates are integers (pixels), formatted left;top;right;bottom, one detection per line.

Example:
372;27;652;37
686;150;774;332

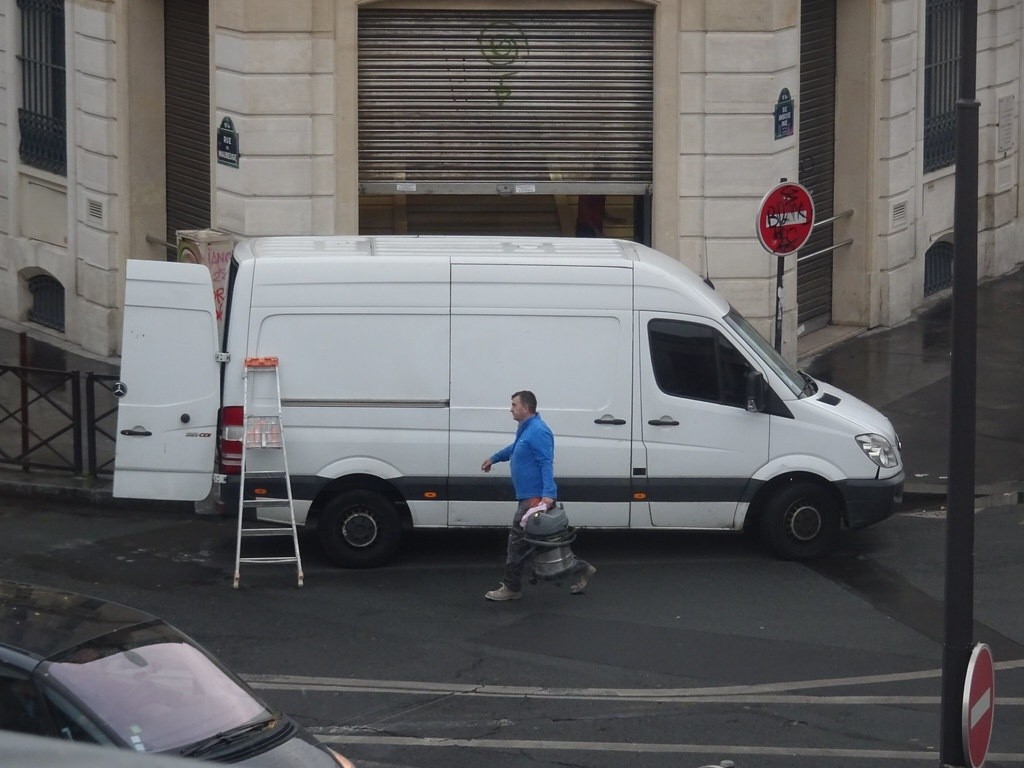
0;581;353;768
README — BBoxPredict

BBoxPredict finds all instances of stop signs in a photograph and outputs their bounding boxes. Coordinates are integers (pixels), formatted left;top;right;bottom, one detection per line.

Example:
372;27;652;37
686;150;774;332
757;182;816;257
962;642;994;766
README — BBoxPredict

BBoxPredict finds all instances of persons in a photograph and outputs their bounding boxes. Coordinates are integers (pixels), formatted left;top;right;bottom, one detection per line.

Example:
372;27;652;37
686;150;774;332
481;391;597;601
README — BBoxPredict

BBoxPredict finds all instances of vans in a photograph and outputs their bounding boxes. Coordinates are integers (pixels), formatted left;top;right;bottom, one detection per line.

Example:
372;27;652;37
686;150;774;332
115;233;907;568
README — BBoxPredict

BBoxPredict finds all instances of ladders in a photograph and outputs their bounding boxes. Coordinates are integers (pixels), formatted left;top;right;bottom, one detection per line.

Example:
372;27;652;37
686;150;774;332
233;356;304;590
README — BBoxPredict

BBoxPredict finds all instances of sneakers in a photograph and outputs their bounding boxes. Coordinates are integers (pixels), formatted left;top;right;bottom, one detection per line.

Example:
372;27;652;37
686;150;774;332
569;564;596;593
484;581;522;600
